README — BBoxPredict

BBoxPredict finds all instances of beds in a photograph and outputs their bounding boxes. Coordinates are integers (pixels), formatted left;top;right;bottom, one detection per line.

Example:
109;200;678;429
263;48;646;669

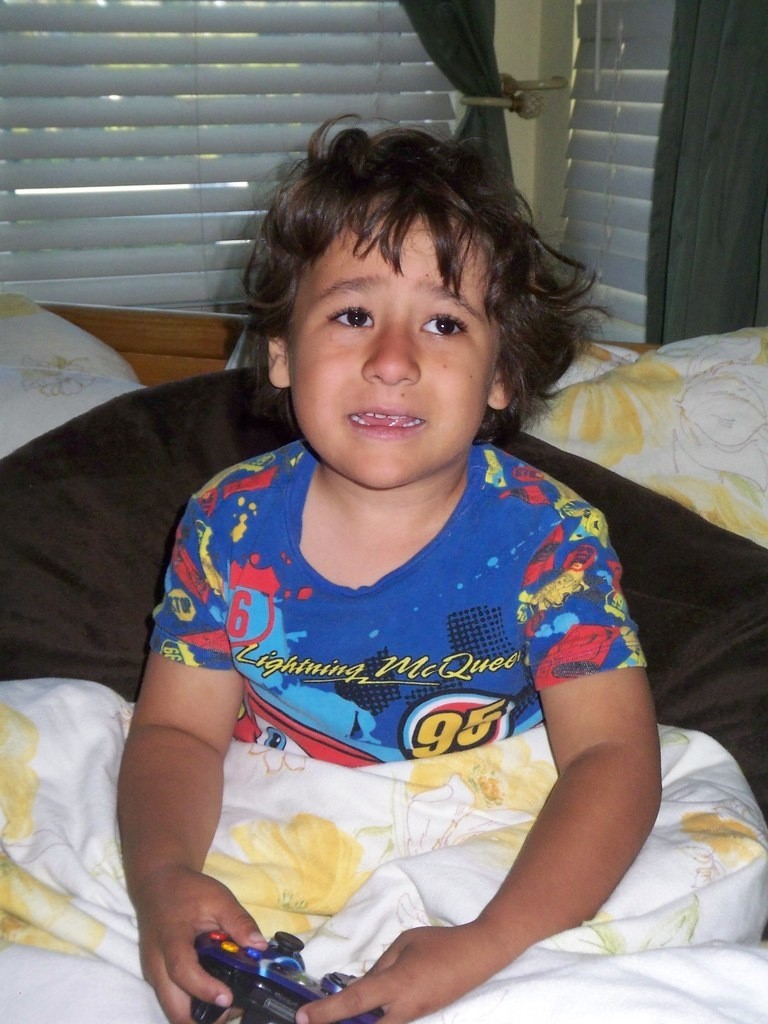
0;292;768;1024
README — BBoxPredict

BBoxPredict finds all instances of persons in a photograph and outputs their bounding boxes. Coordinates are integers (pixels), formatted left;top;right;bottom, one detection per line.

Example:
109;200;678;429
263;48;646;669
117;113;660;1024
